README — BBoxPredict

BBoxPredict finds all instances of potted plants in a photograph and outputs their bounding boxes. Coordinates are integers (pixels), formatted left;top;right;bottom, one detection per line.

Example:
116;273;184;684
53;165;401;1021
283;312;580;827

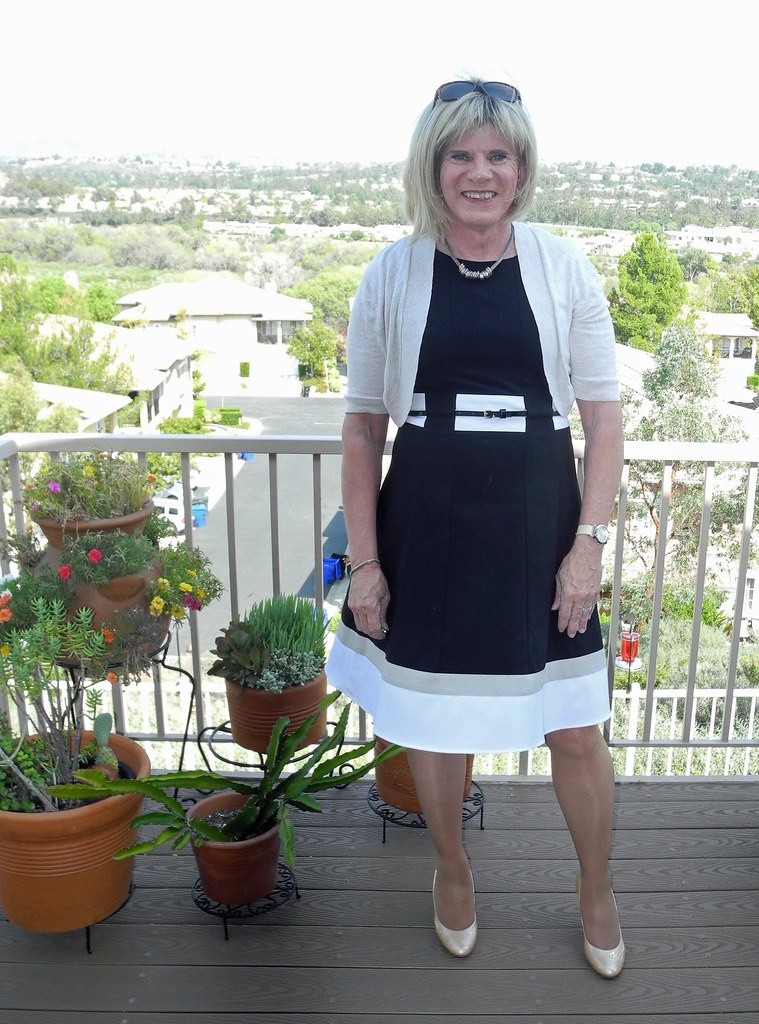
44;688;406;906
206;591;334;755
0;595;151;934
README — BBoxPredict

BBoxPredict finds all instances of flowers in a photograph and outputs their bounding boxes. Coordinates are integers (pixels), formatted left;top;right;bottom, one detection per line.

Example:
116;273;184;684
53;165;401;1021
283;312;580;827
0;442;223;687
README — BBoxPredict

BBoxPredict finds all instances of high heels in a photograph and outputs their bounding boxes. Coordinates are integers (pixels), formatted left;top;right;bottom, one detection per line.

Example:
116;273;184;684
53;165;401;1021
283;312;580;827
578;881;626;980
432;867;479;958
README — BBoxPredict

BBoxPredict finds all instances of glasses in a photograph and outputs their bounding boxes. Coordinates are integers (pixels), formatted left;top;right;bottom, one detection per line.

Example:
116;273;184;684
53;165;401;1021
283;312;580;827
431;81;523;103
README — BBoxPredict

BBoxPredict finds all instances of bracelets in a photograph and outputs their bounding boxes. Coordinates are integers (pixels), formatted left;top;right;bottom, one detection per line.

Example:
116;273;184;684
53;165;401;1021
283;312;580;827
350;559;380;577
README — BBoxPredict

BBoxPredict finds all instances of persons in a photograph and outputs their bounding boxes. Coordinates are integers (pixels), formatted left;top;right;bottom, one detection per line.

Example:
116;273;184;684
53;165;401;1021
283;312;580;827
324;80;624;978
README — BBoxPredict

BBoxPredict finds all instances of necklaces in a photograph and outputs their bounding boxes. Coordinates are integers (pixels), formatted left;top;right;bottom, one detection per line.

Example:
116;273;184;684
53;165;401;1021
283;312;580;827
442;223;514;280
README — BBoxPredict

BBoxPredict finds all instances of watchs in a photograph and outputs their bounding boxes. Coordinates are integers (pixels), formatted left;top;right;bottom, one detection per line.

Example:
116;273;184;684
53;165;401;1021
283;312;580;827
575;524;609;544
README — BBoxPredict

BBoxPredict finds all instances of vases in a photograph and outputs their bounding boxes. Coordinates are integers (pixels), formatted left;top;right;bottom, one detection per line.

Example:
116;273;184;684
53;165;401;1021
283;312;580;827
30;554;172;665
30;497;154;570
374;734;475;814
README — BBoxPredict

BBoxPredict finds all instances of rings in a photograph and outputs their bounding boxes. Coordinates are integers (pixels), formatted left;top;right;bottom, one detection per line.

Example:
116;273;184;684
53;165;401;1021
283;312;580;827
583;605;592;612
